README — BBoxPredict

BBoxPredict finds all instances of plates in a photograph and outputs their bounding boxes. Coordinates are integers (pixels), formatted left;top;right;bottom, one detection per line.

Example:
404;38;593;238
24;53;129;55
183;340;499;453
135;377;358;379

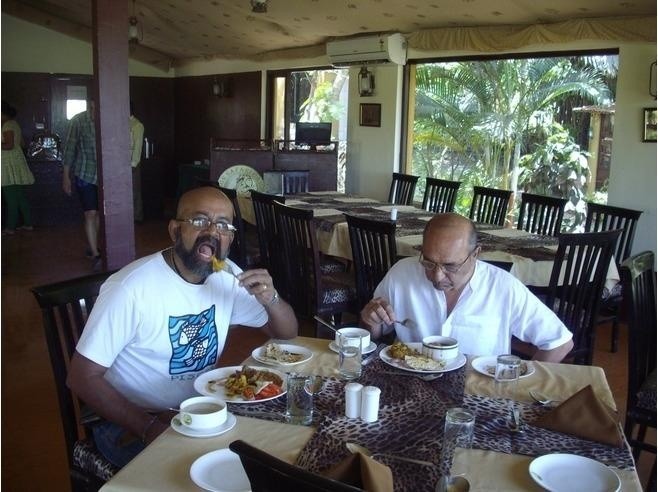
193;366;291;405
250;345;313;367
169;411;238;438
470;355;535;380
188;446;253;492
327;339;378;355
527;452;621;492
378;341;467;375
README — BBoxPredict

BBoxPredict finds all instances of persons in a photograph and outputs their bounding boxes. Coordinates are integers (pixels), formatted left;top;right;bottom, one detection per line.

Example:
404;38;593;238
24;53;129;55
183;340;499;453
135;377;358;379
1;101;36;236
130;102;144;226
359;211;576;367
65;184;300;471
63;110;104;267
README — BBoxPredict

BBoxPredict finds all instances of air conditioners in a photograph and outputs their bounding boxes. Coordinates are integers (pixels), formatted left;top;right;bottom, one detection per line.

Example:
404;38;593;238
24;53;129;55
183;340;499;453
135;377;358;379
325;30;408;68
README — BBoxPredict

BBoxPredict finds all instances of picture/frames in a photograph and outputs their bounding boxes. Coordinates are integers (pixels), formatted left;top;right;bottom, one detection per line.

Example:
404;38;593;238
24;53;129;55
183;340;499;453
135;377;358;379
358;103;382;127
642;107;657;142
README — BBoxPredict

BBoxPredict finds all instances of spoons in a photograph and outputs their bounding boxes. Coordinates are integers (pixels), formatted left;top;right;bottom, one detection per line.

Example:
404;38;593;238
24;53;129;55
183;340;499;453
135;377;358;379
344;441;437;467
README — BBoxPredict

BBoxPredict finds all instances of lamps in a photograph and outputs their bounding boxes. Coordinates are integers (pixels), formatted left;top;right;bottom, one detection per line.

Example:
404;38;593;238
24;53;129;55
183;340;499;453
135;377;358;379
207;74;228;99
357;61;375;97
127;0;146;54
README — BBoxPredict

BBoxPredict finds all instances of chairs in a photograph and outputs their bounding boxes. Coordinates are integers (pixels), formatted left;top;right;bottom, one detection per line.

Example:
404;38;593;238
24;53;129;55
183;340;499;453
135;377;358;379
619;248;656;490
29;270;120;486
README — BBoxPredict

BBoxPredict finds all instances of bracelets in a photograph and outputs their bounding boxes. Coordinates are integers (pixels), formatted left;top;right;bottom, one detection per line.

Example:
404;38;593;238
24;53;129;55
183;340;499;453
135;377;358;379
260;289;279;310
142;416;160;444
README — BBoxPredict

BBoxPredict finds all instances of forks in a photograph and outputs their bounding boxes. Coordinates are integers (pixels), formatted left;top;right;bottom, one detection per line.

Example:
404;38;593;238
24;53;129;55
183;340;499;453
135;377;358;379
221;261;245;284
390;318;418;331
526;391;562;406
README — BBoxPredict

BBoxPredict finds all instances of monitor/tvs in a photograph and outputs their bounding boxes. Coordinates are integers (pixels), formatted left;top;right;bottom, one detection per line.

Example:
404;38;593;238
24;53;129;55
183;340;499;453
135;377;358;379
295;122;332;151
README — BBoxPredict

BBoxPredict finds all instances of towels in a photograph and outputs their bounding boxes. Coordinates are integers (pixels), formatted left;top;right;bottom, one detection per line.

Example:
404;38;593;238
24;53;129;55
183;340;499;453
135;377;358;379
321;447;398;491
530;382;626;449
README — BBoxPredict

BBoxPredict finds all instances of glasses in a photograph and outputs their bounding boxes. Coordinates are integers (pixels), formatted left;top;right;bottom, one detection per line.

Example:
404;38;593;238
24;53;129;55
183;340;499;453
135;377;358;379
418;243;481;271
174;215;239;235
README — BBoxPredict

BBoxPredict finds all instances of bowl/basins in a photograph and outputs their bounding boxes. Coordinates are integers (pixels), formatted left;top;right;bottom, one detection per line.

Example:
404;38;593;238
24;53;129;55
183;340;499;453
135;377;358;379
334;328;370;350
177;395;229;430
421;334;459;362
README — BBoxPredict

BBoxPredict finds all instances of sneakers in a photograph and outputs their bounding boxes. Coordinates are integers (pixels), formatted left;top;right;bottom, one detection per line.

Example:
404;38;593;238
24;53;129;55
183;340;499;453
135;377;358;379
82;247;102;268
1;229;18;238
17;225;35;232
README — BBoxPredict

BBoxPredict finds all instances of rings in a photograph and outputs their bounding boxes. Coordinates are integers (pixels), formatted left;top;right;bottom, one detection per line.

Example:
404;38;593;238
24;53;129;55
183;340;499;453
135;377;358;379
262;284;267;290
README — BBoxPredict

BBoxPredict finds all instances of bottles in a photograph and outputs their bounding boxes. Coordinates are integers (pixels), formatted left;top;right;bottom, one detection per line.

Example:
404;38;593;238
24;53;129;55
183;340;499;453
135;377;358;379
343;383;381;422
390;207;399;221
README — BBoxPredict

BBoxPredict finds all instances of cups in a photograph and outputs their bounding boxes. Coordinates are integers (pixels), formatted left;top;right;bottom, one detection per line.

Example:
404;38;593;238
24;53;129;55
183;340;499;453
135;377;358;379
490;354;520;410
286;371;314;425
441;406;475;478
